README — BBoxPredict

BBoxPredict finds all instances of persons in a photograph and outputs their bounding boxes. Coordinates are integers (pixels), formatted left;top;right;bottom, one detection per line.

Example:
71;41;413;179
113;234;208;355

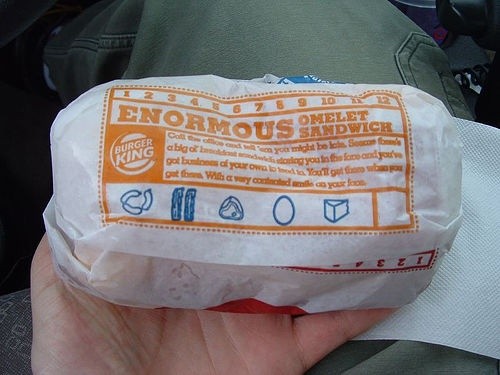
21;0;499;375
